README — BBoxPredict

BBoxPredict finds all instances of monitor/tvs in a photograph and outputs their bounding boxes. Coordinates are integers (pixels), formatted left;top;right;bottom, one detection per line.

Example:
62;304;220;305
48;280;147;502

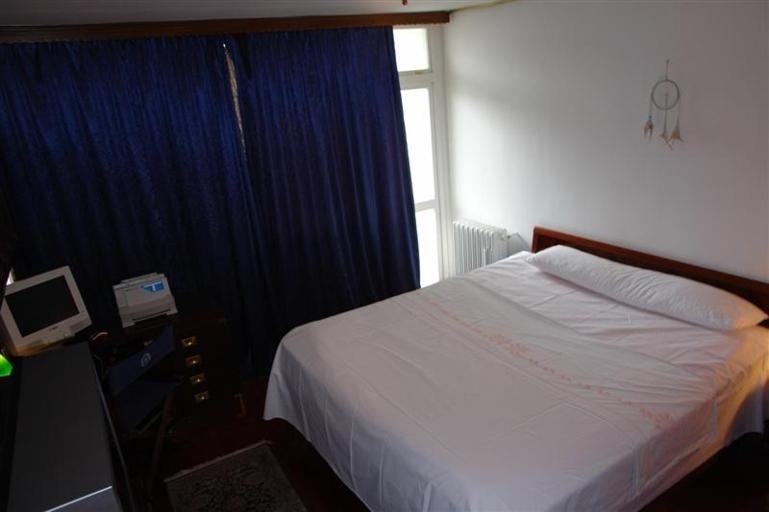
0;341;136;512
0;264;93;358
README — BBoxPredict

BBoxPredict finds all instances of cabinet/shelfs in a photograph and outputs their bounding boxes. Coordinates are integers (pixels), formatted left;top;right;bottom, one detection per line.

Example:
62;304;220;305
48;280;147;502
97;302;246;439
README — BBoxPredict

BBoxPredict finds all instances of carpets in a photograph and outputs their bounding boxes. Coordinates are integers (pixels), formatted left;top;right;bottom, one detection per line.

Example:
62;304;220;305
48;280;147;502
165;439;305;512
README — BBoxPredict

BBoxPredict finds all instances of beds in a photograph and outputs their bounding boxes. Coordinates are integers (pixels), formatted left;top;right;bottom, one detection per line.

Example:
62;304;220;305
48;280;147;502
262;227;769;512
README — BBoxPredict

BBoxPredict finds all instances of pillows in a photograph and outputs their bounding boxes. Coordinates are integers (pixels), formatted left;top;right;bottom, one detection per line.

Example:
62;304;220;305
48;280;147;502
525;244;769;332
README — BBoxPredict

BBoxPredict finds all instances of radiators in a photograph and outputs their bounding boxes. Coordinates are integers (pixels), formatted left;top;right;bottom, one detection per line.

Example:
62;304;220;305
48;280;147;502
453;218;511;274
